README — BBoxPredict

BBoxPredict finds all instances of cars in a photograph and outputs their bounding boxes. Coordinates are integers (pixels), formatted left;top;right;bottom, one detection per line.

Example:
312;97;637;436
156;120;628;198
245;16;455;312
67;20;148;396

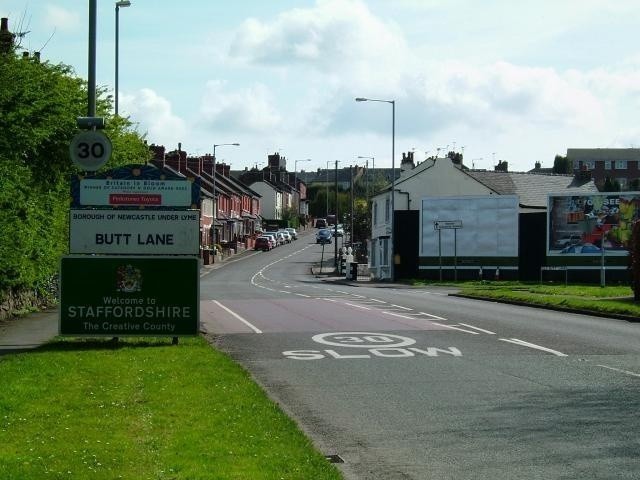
315;229;332;245
254;226;298;252
315;214;344;237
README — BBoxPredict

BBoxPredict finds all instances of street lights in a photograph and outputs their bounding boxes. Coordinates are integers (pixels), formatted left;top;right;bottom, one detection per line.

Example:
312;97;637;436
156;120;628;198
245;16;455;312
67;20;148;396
211;142;241;265
293;156;375;218
354;96;396;209
114;0;132;118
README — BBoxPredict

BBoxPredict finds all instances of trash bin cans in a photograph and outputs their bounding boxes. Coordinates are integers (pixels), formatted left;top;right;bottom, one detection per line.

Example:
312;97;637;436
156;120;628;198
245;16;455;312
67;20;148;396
204;249;218;264
345;262;359;281
